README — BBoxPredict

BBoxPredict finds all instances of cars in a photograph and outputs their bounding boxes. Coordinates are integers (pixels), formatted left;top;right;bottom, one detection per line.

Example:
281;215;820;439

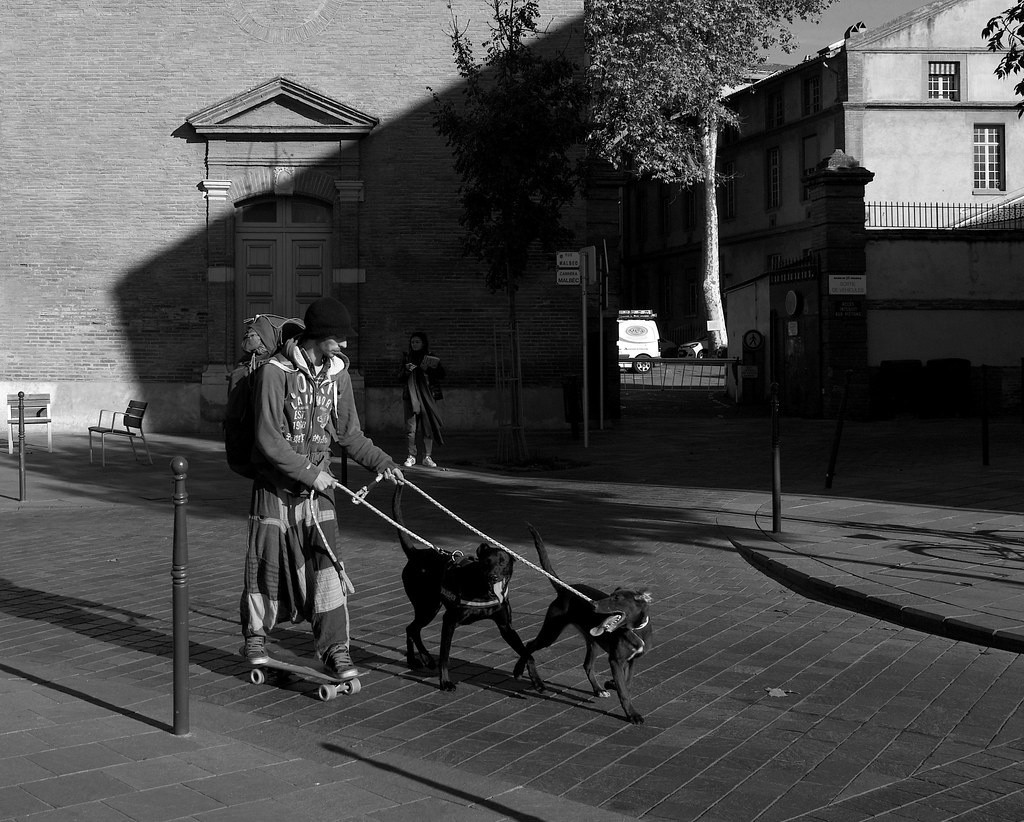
678;337;709;359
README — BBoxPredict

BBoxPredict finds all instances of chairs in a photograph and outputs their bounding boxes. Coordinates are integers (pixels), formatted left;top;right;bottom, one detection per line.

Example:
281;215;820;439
88;400;153;467
7;394;52;454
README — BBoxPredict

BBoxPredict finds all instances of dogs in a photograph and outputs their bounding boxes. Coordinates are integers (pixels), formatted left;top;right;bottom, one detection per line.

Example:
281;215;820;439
391;471;547;694
512;519;655;727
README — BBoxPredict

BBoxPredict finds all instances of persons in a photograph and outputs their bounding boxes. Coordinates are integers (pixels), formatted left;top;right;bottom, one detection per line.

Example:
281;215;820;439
402;331;450;470
227;296;405;681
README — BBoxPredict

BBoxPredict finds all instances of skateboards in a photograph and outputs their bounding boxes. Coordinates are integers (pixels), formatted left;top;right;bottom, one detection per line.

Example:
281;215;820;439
242;653;370;701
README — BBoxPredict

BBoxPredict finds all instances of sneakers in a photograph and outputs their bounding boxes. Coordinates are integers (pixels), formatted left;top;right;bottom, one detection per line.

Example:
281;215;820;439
404;455;416;467
422;456;437;467
322;644;359;679
245;637;269;664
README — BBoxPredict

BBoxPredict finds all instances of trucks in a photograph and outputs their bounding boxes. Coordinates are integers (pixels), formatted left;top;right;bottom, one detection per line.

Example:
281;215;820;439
617;308;664;373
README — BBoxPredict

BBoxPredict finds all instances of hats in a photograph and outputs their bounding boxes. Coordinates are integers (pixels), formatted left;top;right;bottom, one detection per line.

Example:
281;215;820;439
304;297;352;339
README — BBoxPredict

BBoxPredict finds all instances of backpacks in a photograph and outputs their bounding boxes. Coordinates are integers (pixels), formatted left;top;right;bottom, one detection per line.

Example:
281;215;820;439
225;355;293;481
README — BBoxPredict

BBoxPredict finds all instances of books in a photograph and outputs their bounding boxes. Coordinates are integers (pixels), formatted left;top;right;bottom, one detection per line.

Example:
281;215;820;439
423;355;441;368
